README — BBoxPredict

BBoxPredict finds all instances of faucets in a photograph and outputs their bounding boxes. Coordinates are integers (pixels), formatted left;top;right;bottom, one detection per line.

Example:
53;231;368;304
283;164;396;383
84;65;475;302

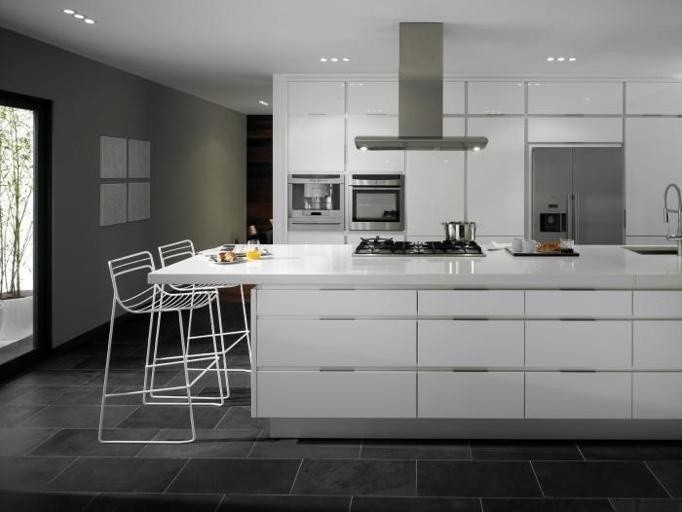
662;183;682;257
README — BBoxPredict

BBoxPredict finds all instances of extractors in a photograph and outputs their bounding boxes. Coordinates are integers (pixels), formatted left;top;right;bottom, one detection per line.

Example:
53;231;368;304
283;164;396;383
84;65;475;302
354;21;488;151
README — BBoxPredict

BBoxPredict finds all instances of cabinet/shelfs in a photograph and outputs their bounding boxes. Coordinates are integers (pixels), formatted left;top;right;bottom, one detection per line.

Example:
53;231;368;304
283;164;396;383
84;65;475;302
285;82;344;172
418;288;524;420
525;291;633;419
633;291;682;420
527;81;624;148
250;289;418;419
345;82;404;172
624;80;682;244
404;82;465;236
465;82;527;236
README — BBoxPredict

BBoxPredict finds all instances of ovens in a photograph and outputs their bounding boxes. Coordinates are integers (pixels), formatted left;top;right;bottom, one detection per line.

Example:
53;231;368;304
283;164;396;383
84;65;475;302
349;173;405;231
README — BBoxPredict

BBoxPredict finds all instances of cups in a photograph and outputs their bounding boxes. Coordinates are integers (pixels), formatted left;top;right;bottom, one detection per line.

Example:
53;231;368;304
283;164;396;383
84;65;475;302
559;237;574;254
522;239;541;253
511;238;522;252
246;239;261;260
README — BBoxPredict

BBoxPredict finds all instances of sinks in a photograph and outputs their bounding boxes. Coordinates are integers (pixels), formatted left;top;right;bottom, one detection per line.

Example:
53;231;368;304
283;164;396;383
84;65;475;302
622;246;678;255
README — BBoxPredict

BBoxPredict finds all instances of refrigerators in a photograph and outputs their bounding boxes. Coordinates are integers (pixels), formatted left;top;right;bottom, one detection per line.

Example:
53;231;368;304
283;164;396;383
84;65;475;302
531;147;626;246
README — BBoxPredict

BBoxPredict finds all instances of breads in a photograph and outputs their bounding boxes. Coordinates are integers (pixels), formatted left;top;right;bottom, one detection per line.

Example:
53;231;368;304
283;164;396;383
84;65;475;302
216;253;238;263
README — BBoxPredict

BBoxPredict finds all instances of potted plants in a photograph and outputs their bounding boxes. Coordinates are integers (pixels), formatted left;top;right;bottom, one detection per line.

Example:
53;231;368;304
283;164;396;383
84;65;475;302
0;106;36;341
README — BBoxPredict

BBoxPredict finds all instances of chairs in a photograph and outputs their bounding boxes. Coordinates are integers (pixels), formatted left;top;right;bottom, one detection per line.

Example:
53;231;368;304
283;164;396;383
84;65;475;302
142;239;252;407
98;250;227;445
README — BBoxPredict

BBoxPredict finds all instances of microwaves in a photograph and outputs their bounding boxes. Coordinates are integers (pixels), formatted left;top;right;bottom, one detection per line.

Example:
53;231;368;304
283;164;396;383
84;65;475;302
286;173;344;218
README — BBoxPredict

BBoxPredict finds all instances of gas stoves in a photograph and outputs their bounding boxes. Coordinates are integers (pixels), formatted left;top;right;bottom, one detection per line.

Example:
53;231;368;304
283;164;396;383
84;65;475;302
354;235;487;257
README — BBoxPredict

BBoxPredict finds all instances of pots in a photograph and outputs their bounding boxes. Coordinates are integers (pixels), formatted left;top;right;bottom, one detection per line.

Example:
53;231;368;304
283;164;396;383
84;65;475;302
442;221;477;245
